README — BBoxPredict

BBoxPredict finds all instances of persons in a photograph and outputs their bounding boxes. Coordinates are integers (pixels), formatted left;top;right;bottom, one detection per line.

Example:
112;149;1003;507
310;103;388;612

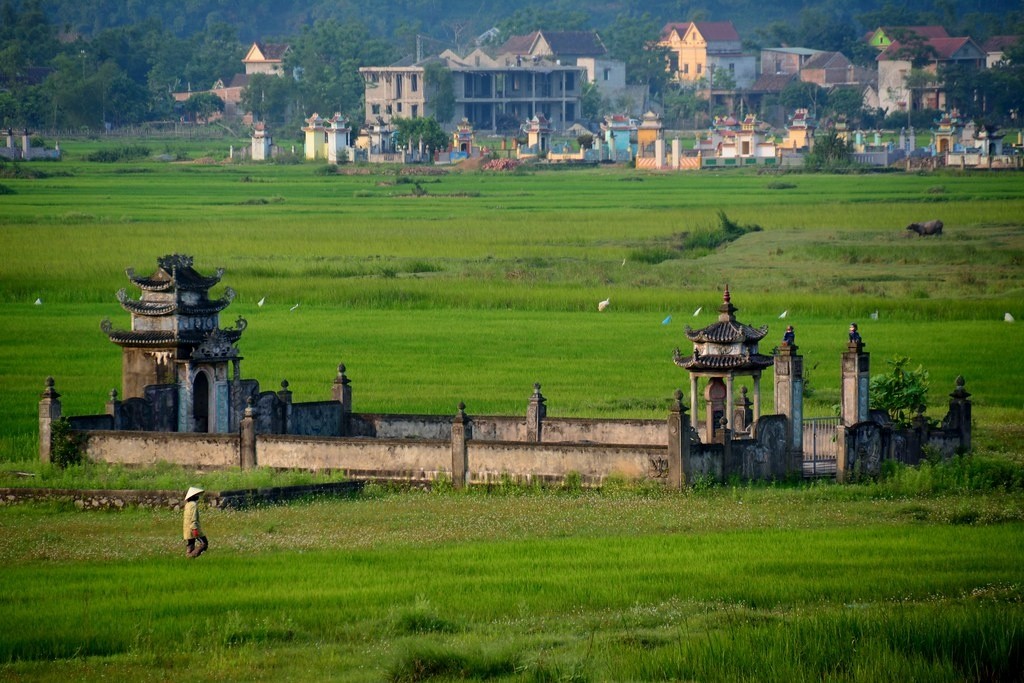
782;326;795;347
183;486;208;558
847;322;861;343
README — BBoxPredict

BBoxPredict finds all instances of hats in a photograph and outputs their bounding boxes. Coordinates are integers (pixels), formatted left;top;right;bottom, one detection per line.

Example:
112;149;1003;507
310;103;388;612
185;487;204;500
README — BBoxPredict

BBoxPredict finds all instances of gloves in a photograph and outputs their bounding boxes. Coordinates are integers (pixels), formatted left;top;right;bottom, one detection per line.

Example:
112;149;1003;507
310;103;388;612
193;530;198;536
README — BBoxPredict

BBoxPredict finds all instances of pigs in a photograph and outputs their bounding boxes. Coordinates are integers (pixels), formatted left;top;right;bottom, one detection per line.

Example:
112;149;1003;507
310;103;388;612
906;220;944;238
